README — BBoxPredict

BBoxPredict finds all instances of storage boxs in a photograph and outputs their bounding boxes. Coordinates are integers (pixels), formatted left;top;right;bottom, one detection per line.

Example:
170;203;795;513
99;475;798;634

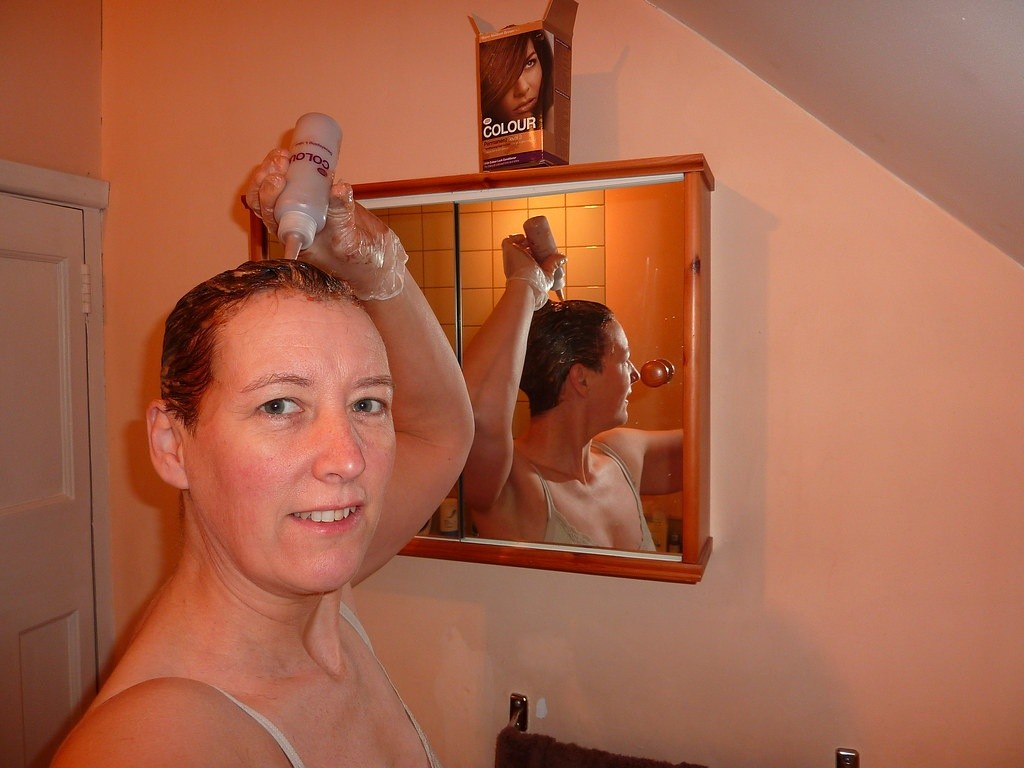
472;0;579;172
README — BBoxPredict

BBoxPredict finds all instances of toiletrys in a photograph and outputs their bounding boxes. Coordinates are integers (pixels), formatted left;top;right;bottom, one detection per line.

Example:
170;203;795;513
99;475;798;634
667;533;681;553
523;216;566;301
272;112;343;261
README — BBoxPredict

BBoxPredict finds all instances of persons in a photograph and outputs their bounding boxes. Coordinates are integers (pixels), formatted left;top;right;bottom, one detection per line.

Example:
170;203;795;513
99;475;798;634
480;30;555;135
462;233;683;552
49;149;475;768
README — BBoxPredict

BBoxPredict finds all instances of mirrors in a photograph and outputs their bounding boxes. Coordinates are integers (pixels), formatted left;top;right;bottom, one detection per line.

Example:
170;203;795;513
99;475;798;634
240;153;715;583
